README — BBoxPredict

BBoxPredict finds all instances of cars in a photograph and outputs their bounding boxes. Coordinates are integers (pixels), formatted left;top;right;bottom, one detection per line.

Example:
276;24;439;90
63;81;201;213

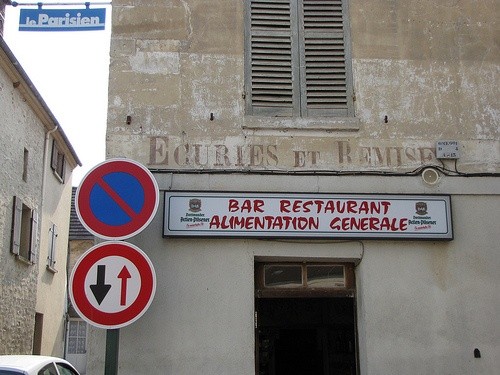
0;355;80;375
